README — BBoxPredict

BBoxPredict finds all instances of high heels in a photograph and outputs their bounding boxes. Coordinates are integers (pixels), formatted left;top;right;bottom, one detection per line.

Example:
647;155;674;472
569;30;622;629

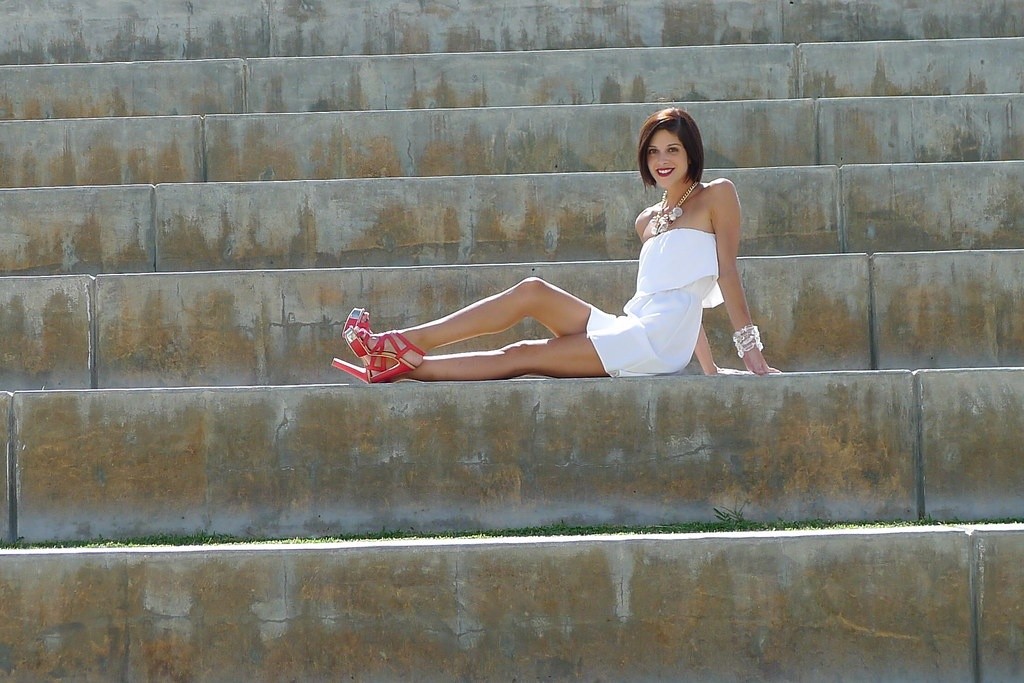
343;325;426;383
332;307;373;384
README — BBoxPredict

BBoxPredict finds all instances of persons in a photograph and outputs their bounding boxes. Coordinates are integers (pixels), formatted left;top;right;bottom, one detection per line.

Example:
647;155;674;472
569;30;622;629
331;104;783;383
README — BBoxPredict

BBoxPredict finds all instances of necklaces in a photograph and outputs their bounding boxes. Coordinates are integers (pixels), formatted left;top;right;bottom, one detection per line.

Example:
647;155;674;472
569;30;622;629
649;181;699;236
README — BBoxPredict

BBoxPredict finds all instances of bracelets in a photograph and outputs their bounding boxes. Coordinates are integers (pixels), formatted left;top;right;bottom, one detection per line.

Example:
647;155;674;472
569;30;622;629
732;325;764;359
